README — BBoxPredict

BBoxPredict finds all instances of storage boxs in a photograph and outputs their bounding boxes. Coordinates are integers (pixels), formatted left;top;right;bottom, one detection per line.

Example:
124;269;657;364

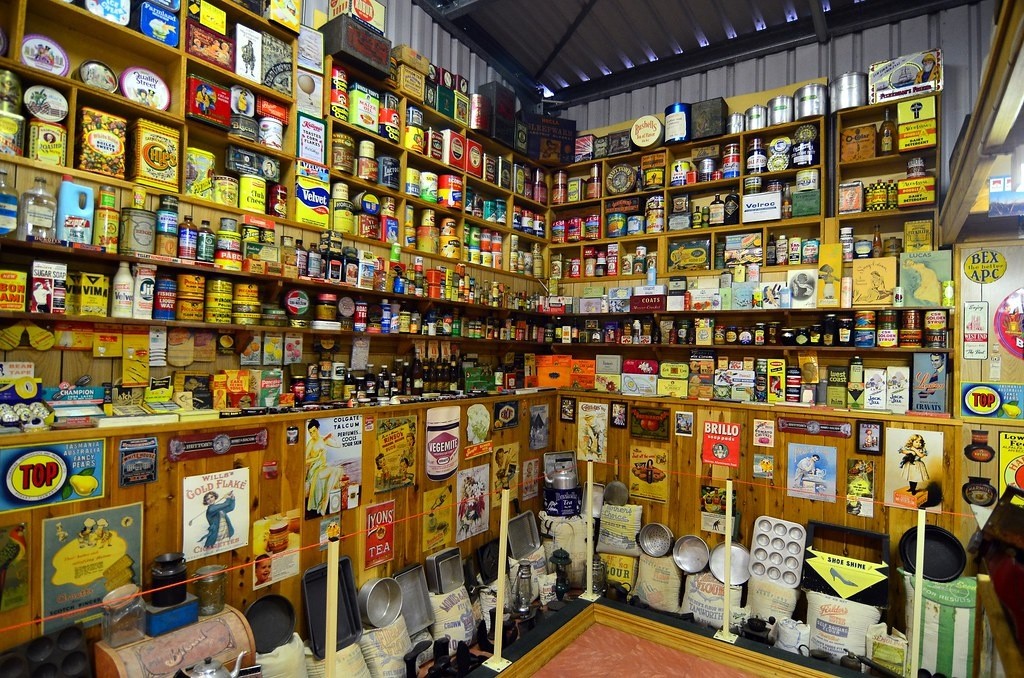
695;327;712;345
724;247;762;264
595;354;622;374
389;42;429;75
863;367;886;410
656;378;687;397
185;73;231;131
579;298;601;313
514;109;576;166
102;276;108;316
629;294;665;313
667;239;709;272
78;271;104;316
633;284;668;295
725;232;761;250
886;366;909;414
394;63;425;103
621;372;658;395
65;271;80;313
594;373;621;391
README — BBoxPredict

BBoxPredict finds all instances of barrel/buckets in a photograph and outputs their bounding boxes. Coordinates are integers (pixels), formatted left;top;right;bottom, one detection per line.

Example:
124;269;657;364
56;175;94;246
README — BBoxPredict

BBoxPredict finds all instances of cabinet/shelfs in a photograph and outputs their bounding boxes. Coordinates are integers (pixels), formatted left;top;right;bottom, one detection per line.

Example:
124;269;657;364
0;1;958;663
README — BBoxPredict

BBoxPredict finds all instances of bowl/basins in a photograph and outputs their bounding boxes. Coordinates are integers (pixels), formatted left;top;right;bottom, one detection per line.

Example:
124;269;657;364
673;534;709;573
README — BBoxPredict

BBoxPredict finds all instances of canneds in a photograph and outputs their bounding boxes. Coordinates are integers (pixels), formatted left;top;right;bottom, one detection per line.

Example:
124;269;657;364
213;64;547;281
549;134;819;278
156;273;258;324
213;217;276;270
291;359;346;401
312;245;696;345
92;185;179;257
714;309;948;350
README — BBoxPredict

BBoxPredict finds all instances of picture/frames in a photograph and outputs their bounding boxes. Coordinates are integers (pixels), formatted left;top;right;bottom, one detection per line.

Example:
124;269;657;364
854;418;884;456
610;400;628;428
674;410;693;437
559;396;576;423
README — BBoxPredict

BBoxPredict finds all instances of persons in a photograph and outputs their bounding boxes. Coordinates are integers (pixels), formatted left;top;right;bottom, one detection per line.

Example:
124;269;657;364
524;462;535;493
495;448;505;466
702;487;727;514
254;554;272;586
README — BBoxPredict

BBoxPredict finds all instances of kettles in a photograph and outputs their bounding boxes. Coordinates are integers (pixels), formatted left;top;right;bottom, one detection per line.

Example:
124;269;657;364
543;457;577;489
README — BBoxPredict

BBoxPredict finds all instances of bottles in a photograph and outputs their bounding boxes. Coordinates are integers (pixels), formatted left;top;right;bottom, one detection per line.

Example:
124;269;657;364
864;179;898;211
989;343;1002;380
877;108;898;156
179;202;855;347
709;188;740;227
110;260;134;318
17;177;57;244
0;168;20;238
873;224;882;257
782;183;792;219
344;354;525;405
